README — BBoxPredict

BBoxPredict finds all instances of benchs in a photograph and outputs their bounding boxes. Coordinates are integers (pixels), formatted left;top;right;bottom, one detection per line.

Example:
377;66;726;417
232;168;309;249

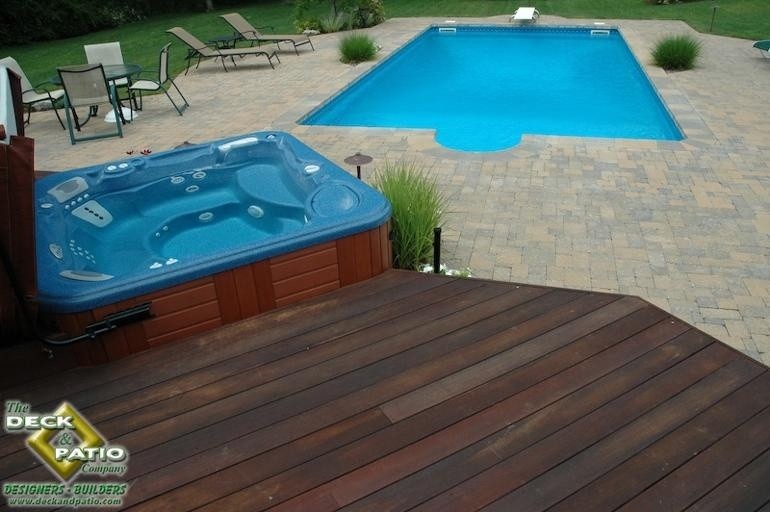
509;5;540;23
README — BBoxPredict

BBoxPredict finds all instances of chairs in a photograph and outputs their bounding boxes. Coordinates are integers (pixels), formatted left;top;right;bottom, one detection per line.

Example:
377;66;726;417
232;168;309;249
3;40;188;145
165;12;315;76
